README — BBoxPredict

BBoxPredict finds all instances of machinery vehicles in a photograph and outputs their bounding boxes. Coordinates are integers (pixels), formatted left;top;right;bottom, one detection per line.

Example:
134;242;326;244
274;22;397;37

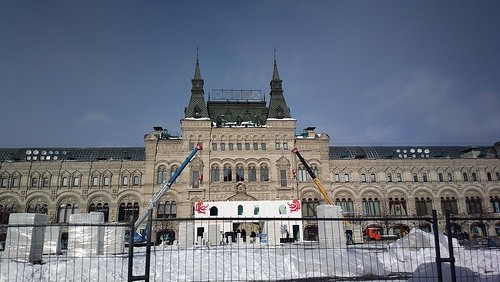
124;144;203;246
291;148;381;245
